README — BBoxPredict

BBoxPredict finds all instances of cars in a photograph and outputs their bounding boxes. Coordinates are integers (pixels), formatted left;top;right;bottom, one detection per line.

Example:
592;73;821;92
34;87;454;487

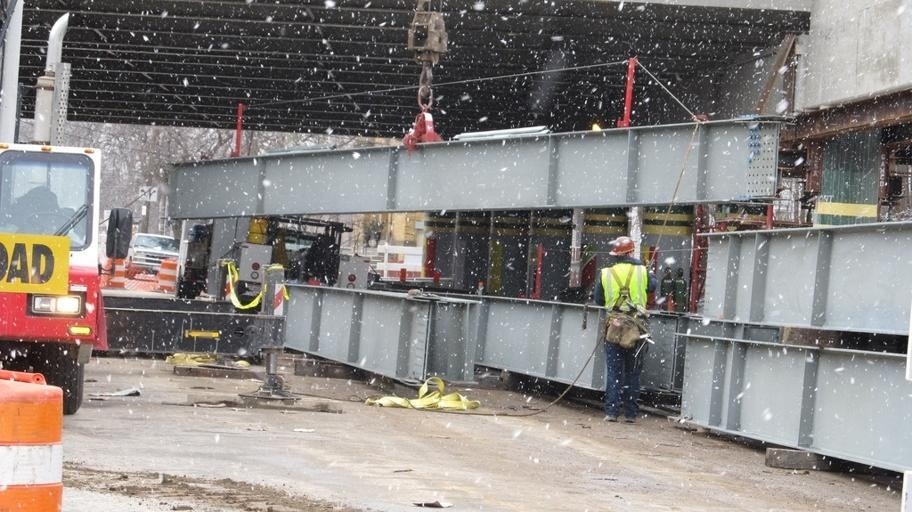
124;233;180;276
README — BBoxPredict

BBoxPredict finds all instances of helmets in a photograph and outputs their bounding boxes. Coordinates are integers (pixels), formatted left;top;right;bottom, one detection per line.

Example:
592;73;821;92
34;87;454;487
609;236;635;256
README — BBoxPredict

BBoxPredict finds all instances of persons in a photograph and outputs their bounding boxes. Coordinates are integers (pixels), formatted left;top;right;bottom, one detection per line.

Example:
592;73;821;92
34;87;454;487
597;236;658;420
671;267;687;313
661;268;675;299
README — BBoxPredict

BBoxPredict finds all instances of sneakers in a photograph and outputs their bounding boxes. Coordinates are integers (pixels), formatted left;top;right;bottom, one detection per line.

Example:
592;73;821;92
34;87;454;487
603;414;636;423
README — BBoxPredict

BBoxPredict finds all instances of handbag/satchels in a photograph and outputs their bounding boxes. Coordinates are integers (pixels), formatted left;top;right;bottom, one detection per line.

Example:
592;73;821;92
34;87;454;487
606;313;640;349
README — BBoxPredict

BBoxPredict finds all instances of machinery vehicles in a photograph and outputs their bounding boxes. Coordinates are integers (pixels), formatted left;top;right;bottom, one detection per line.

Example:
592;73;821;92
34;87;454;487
0;143;301;414
